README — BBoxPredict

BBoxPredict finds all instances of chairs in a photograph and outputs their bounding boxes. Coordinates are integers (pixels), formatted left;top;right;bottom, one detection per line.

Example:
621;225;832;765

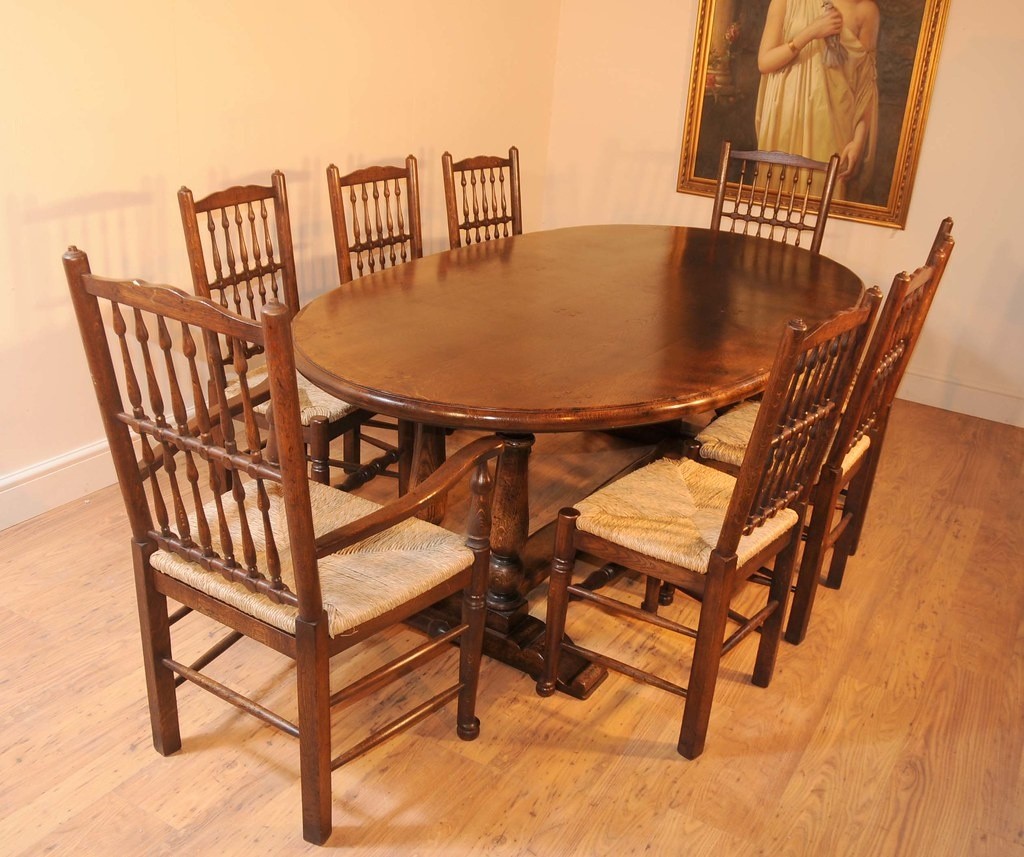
534;284;883;759
442;146;523;249
62;242;512;846
326;154;423;286
657;217;956;645
709;139;840;253
178;168;416;499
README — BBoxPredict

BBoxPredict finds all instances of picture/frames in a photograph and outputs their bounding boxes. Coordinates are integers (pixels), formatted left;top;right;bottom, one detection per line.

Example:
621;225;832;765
677;0;952;231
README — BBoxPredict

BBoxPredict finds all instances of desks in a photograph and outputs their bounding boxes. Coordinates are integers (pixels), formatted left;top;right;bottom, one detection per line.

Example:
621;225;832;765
292;221;867;699
